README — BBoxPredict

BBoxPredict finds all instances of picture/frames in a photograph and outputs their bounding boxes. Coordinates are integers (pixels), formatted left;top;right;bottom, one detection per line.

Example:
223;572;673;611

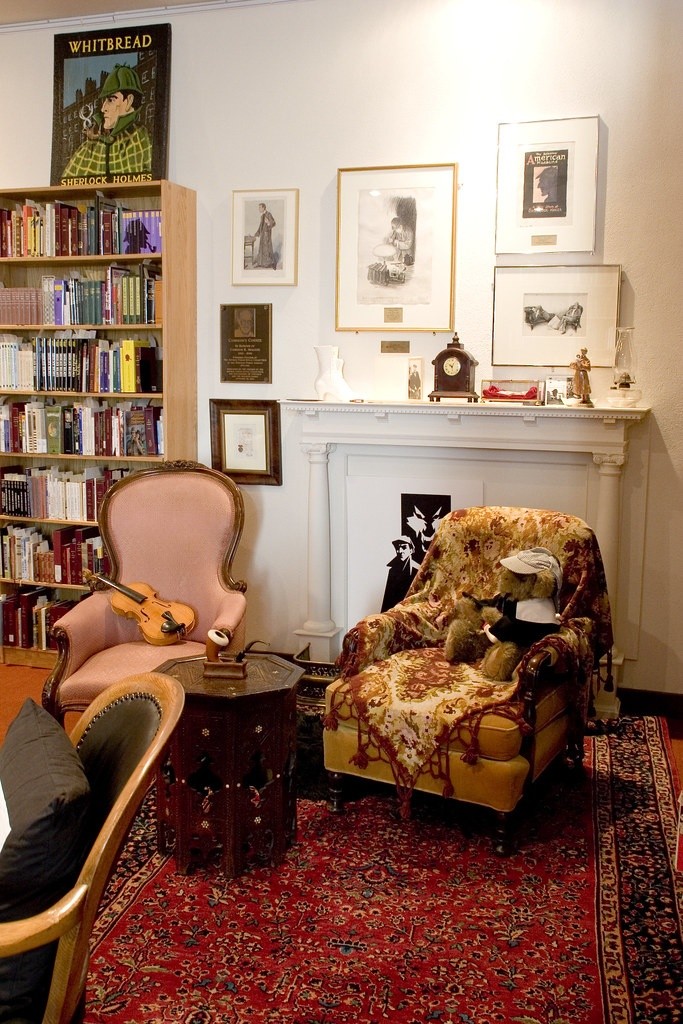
209;398;283;486
50;22;173;187
335;163;458;334
408;357;423;404
491;264;622;369
231;187;300;286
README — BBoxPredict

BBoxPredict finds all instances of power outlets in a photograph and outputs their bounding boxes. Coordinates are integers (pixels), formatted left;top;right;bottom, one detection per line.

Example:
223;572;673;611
380;340;410;353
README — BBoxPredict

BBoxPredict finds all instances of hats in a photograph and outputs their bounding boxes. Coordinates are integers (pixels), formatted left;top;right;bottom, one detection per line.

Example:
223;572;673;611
500;546;563;592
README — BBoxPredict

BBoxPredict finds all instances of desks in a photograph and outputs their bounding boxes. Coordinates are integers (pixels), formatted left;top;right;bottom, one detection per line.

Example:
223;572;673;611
148;651;307;878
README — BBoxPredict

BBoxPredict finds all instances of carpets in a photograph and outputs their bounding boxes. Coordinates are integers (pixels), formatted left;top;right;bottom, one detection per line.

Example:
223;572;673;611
82;694;683;1024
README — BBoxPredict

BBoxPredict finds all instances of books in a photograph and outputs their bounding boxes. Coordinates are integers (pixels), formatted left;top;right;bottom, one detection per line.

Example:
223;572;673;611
0;262;164;325
0;526;103;585
0;587;93;652
0;333;163;393
0;464;131;522
0;397;164;456
0;195;162;258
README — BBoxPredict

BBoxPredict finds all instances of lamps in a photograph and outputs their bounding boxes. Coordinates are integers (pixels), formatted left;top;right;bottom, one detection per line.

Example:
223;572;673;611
610;325;642;409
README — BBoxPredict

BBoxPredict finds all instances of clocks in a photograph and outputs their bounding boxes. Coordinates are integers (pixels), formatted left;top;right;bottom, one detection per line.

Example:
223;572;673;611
428;331;480;403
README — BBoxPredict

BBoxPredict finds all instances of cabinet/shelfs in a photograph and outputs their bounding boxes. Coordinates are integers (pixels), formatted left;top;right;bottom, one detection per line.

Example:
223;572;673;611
0;180;196;671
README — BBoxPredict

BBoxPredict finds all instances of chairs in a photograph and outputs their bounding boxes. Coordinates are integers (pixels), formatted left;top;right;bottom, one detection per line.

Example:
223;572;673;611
321;505;615;855
0;669;184;1024
41;458;246;730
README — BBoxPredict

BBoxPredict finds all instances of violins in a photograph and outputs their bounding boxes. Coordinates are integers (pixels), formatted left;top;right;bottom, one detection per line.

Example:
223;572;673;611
82;567;196;645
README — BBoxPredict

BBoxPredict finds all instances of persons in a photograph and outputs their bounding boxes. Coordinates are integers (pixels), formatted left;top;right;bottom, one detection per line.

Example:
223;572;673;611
135;429;147;455
234;309;254;337
569;348;593;404
126;427;141;456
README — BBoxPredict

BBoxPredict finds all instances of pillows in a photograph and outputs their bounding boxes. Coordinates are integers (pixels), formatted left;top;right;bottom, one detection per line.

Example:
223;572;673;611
0;698;91;1013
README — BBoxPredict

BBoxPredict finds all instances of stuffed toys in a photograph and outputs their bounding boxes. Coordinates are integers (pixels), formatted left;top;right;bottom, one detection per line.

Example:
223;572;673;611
445;548;562;680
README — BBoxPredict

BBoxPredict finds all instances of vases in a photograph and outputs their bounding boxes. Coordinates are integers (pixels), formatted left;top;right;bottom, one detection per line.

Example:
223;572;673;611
315;345;346;402
